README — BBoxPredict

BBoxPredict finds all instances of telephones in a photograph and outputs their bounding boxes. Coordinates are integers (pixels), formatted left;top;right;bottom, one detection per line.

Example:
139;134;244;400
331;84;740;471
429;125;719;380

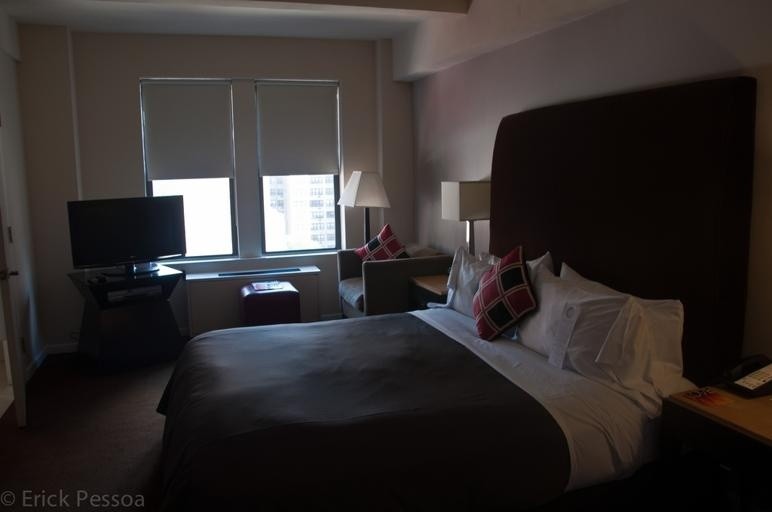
726;352;772;399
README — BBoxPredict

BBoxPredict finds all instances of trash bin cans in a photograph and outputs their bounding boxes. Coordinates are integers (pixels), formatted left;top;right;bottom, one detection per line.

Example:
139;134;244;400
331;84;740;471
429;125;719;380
72;342;113;395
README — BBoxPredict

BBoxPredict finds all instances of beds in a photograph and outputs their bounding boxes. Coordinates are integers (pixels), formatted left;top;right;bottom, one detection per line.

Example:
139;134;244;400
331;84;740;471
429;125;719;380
154;71;754;509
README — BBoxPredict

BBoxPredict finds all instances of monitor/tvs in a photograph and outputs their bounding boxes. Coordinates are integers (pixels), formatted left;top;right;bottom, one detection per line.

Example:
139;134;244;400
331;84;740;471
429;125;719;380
67;195;187;276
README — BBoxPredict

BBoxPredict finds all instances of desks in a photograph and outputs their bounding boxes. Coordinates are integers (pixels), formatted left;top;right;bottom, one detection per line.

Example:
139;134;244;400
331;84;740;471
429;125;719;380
186;266;322;334
71;266;184;369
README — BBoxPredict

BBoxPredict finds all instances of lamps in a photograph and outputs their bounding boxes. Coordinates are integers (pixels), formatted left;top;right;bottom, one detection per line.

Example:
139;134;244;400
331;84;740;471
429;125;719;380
337;170;391;248
437;181;490;256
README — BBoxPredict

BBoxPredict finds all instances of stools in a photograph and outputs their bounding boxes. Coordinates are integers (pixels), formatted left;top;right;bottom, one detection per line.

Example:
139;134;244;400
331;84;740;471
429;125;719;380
237;279;303;325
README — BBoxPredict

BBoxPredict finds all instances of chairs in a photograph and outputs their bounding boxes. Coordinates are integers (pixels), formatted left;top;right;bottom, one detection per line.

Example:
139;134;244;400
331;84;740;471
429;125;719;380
337;245;451;318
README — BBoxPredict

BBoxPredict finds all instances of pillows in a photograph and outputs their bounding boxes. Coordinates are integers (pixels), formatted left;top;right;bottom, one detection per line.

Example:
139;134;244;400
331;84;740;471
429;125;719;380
426;245;500;321
357;226;411;259
559;262;685;379
473;245;547;342
475;250;551;284
515;262;662;419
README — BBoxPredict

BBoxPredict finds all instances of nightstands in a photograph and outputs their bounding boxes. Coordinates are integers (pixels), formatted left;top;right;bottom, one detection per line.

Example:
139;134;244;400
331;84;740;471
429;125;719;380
661;384;772;512
412;273;461;308
